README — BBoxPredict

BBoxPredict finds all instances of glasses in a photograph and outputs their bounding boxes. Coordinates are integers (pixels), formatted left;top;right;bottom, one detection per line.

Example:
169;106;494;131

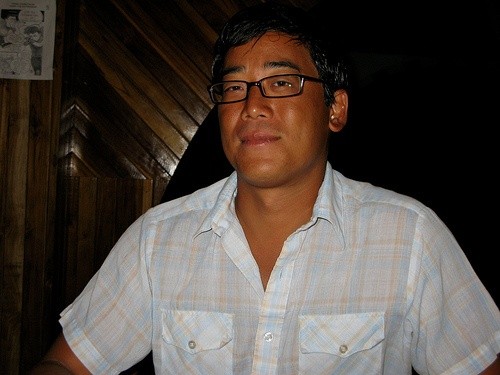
207;73;323;105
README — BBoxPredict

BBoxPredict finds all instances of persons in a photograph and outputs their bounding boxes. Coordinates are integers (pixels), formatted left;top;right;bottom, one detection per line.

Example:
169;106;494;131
23;0;500;374
